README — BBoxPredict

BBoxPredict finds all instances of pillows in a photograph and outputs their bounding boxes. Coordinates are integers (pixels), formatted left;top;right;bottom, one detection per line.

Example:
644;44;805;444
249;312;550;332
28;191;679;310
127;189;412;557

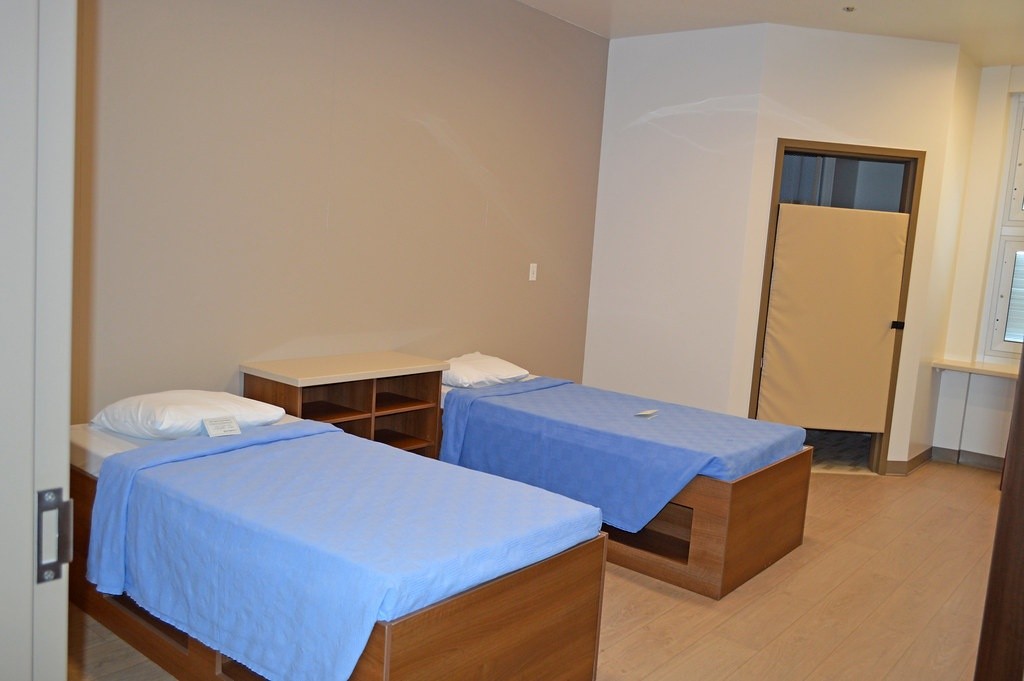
90;389;286;441
441;353;529;388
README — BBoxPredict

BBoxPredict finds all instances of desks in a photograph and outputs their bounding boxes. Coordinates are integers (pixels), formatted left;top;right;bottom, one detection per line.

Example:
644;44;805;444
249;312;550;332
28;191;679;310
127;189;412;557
931;355;1021;380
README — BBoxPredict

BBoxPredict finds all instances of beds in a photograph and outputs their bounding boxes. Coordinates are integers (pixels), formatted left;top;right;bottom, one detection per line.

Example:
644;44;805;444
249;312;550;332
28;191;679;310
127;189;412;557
66;423;608;681
440;374;814;602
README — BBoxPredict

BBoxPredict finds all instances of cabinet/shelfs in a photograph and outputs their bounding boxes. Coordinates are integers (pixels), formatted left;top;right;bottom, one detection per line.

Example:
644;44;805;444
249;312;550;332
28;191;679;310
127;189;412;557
237;351;451;461
